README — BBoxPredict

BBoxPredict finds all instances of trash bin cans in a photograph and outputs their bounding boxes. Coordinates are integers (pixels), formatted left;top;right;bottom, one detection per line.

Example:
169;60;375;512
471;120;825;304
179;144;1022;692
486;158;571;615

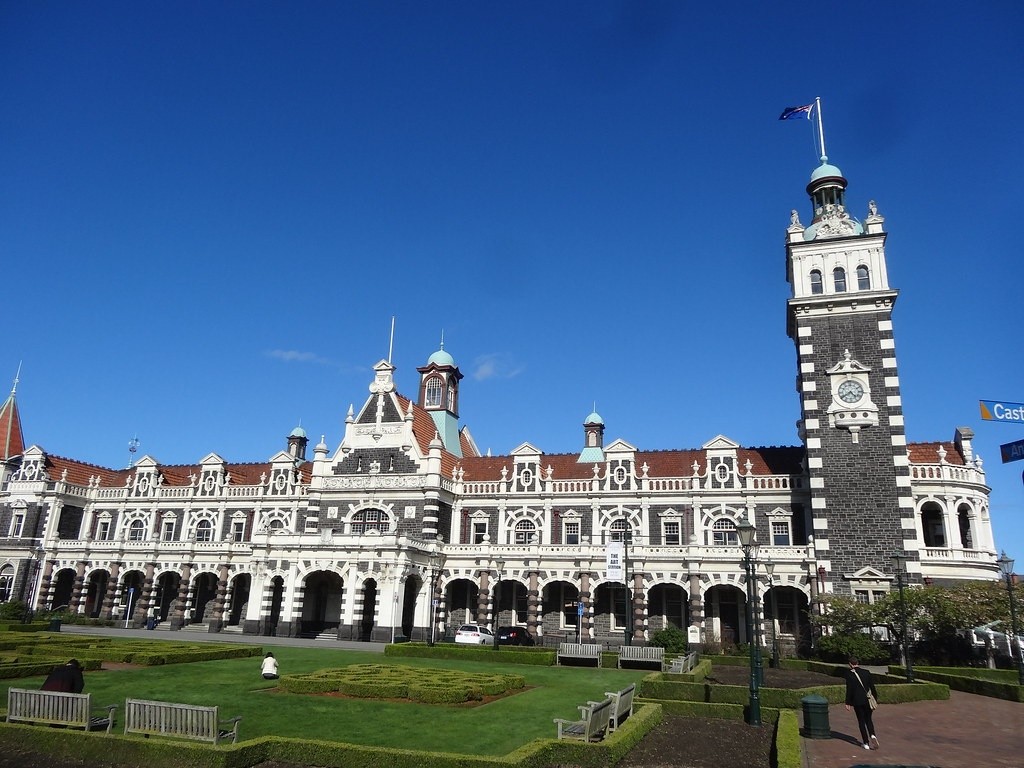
928;650;941;666
445;637;455;643
147;617;154;630
394;636;410;643
800;695;832;739
49;618;62;632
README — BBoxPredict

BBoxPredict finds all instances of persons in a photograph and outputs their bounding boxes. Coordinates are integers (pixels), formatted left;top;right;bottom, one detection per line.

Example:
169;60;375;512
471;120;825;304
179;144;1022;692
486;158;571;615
260;652;279;678
39;658;85;729
845;656;879;750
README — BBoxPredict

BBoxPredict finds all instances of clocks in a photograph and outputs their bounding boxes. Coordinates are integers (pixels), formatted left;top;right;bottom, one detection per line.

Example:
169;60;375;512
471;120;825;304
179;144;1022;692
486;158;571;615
832;378;867;404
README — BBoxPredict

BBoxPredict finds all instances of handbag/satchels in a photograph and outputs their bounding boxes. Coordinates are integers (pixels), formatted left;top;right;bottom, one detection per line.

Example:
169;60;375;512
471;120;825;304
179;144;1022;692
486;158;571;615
867;689;878;710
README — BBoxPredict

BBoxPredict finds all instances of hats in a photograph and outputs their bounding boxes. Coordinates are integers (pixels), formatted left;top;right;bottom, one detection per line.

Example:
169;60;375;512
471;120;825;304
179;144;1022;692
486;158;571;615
66;659;82;670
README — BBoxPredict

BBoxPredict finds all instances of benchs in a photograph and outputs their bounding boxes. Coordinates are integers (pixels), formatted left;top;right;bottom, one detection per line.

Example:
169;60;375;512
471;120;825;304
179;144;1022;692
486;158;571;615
122;697;243;745
618;646;667;669
676;651;698;667
556;643;604;667
6;686;119;734
664;655;692;673
588;682;636;729
554;698;614;742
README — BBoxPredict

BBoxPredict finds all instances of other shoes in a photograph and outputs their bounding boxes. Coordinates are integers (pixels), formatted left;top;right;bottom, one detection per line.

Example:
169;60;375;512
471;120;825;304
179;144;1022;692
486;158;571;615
871;734;879;749
861;743;869;750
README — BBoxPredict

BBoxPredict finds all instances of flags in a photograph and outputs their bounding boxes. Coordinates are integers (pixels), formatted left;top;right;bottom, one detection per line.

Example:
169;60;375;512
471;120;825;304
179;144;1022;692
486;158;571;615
778;103;814;120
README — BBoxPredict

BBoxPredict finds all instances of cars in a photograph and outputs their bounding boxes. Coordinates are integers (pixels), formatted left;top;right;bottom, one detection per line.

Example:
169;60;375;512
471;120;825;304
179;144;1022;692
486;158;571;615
496;626;535;646
454;624;494;645
972;619;1024;644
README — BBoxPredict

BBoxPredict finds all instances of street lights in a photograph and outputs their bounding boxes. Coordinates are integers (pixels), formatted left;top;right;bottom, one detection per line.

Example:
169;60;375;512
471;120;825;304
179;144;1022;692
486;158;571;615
889;548;916;683
734;511;762;725
996;549;1024;686
749;539;766;686
